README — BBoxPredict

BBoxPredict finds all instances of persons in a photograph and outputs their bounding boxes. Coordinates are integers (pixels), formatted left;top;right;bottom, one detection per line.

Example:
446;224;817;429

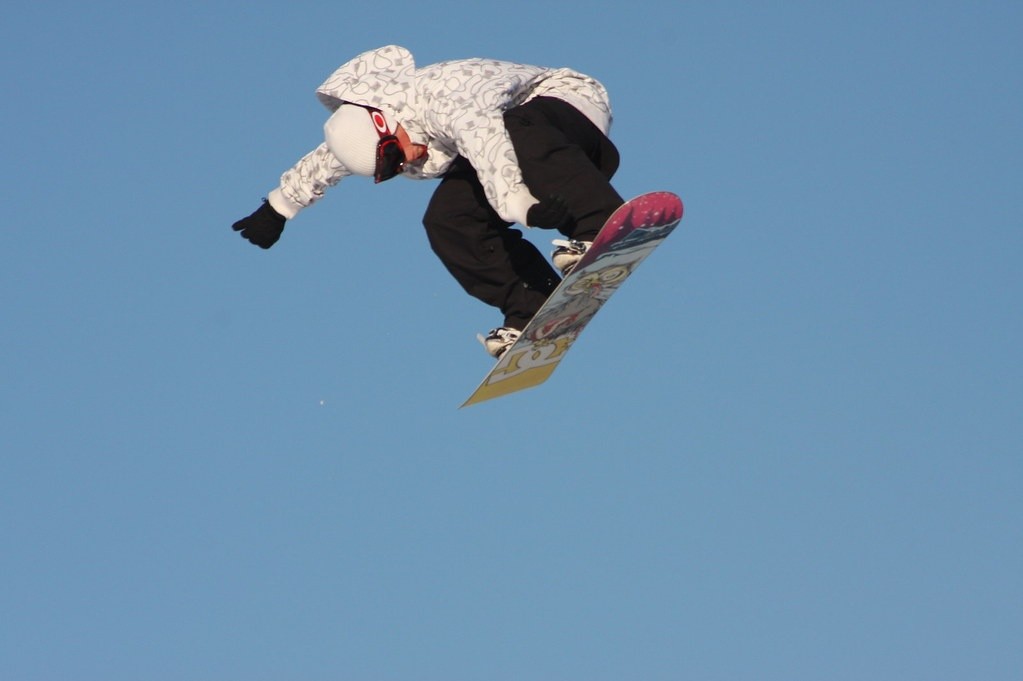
232;44;627;359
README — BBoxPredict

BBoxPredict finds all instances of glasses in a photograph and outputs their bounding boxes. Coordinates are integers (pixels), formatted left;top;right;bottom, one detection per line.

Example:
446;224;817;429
374;136;404;183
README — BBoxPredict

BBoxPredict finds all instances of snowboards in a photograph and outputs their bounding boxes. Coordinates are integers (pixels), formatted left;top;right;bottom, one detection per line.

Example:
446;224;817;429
456;189;684;411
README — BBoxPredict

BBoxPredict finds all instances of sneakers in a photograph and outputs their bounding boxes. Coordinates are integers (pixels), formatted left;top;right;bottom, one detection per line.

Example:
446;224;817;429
550;236;593;275
485;327;521;358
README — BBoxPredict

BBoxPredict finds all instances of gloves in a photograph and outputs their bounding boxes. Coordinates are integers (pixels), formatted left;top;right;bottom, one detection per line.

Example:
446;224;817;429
527;203;572;236
231;197;286;249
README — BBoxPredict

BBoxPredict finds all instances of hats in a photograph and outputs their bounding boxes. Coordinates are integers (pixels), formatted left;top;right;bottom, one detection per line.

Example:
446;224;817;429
324;103;398;178
